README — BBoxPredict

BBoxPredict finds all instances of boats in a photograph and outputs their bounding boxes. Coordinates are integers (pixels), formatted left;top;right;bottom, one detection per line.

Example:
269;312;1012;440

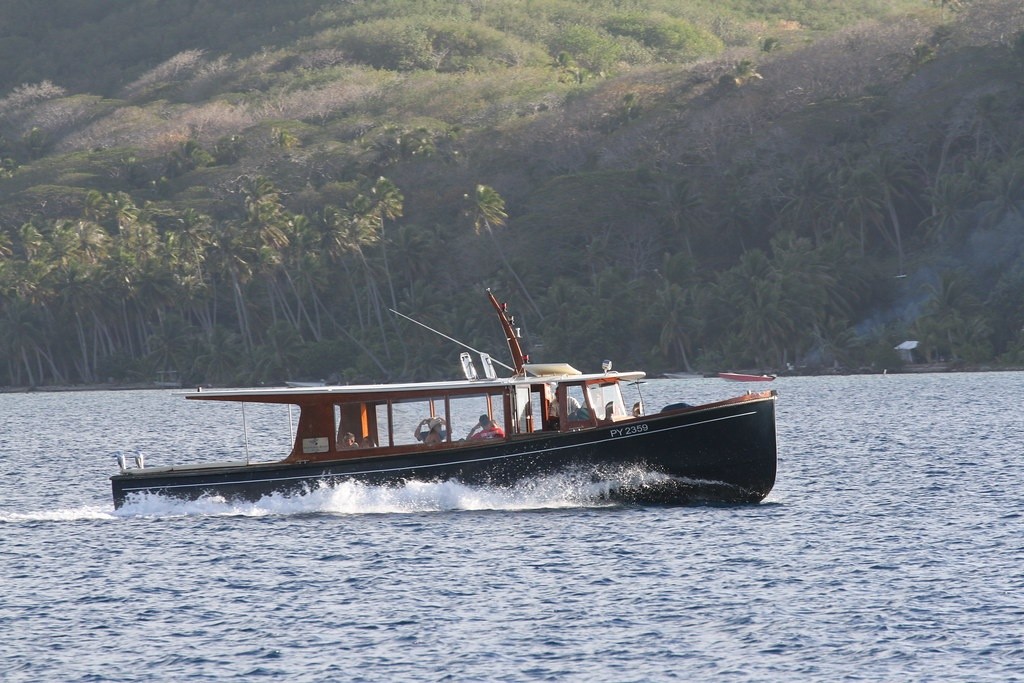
111;290;777;511
718;372;777;384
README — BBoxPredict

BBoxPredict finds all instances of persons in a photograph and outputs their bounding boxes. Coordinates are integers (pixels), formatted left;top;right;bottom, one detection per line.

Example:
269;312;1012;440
548;387;581;420
414;417;453;445
466;414;505;440
361;436;374;448
336;432;358;449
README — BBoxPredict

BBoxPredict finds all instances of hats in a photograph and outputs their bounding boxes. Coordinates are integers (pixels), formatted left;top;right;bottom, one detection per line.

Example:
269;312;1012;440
429;418;441;435
